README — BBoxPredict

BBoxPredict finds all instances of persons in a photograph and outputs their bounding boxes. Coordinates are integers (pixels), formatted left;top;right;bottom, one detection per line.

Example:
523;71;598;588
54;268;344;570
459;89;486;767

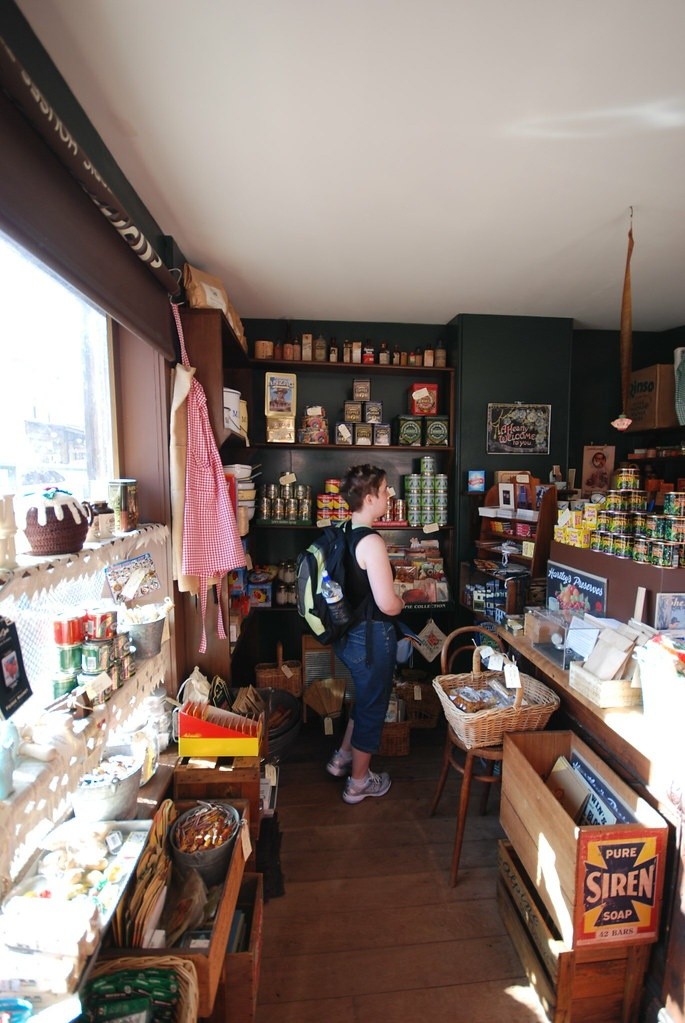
326;465;405;804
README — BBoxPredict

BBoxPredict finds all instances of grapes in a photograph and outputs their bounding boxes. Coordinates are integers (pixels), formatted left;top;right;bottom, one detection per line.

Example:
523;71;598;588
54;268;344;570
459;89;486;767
556;596;587;613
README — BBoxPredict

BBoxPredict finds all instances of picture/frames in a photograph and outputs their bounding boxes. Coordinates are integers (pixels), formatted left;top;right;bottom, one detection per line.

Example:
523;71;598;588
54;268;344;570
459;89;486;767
485;402;552;457
498;482;515;510
263;371;296;417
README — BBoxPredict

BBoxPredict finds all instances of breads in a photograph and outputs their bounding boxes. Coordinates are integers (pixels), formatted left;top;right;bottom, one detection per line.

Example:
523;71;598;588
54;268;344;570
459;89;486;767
52;826;125;897
448;687;486;712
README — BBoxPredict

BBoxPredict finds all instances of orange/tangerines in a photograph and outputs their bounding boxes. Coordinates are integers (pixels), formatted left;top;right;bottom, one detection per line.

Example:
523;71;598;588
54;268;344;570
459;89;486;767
560;587;580;601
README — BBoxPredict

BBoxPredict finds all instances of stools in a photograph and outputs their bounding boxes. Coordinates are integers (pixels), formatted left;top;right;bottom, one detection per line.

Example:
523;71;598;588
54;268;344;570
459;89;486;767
429;726;514;887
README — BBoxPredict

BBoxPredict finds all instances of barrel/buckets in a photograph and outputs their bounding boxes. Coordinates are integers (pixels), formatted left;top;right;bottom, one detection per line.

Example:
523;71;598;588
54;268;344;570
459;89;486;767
170;803;240;888
69;742;145;821
127;609;166;660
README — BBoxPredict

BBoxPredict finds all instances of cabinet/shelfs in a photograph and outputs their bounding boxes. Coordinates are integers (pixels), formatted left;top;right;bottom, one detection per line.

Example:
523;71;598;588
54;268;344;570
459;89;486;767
477;482;555;577
245;364;455;612
0;523;171;894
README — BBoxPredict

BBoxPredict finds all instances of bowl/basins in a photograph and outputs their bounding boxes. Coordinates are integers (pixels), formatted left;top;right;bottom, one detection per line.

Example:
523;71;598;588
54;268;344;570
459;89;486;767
223;464;256;520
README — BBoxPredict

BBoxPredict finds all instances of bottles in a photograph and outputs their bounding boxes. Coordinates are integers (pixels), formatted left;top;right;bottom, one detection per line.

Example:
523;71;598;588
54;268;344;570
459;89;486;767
379;342;390;365
314;332;351;362
274;338;282;361
321;570;350;624
408;339;446;367
293;338;301;360
393;345;400;365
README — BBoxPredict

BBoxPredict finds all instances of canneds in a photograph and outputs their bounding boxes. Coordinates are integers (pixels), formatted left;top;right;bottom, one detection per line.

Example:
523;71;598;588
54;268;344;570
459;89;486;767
589;468;685;571
256;471;312;521
316;478;354;521
54;606;136;705
646;445;656;458
276;561;298;606
379;455;448;526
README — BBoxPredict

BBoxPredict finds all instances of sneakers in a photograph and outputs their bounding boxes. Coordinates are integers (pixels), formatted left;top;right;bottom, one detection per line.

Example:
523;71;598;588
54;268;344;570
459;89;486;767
326;750;351;776
342;769;392;804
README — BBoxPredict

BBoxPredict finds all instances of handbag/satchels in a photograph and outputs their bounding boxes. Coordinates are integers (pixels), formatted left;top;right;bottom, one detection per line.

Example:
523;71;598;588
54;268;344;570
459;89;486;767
412;619;447;662
396;639;412;663
176;667;210;704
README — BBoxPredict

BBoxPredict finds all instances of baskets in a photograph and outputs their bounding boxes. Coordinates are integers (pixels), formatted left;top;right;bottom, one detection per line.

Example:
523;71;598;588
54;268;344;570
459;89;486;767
524;613;565;644
569;661;641;708
87;955;198;1023
377;720;412;756
433;645;561;749
256;643;303;698
26;503;89;555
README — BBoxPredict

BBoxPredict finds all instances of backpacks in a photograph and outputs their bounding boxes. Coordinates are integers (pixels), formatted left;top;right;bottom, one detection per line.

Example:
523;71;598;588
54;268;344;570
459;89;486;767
295;520;373;646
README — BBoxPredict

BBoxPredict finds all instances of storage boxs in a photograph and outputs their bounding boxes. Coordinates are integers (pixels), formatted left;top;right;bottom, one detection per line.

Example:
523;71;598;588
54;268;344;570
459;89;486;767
226;559;275;608
532;611;603;671
332;374;449;447
466;580;517;625
623;363;677;433
503;729;667;947
100;793;264;1023
497;840;652;1022
